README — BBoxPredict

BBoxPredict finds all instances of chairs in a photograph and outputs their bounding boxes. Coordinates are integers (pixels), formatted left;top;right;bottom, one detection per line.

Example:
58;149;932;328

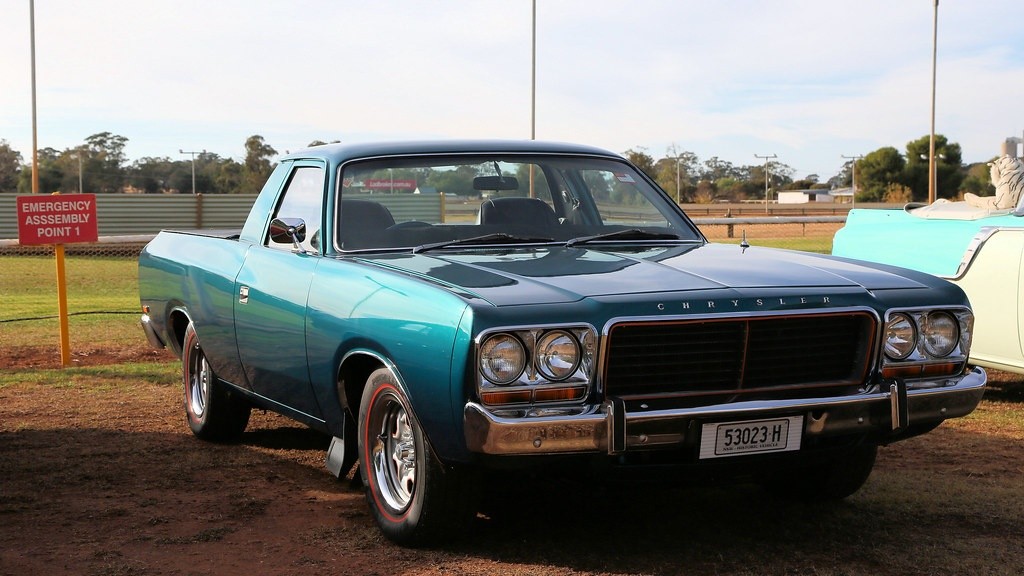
338;196;562;239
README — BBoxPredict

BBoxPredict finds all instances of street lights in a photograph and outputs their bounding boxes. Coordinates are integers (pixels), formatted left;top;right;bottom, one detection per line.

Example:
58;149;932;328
920;154;946;205
179;149;207;194
753;153;778;215
840;153;864;211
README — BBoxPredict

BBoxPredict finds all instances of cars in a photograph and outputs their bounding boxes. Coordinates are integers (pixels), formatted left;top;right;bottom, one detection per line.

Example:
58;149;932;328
134;136;989;552
